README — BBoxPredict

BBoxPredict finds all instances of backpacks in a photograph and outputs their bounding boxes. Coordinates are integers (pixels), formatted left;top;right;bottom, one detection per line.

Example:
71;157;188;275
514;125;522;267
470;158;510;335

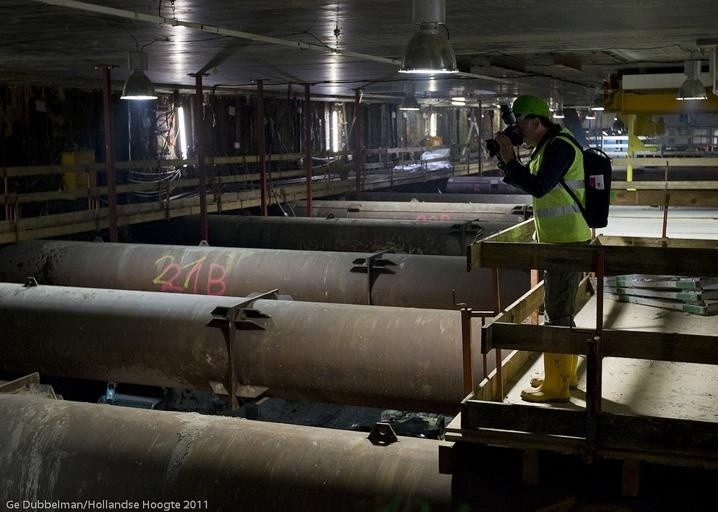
543;133;611;228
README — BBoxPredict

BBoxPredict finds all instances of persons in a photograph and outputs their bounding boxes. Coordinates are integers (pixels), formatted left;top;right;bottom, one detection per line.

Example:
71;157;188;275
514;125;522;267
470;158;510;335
491;94;593;402
613;116;624;152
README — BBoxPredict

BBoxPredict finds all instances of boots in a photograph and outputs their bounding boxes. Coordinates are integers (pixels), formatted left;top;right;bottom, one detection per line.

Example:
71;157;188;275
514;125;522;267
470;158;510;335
521;352;578;403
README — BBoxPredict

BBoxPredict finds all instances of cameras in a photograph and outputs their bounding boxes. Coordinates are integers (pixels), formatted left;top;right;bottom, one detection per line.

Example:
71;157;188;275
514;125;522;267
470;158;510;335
485;101;522;157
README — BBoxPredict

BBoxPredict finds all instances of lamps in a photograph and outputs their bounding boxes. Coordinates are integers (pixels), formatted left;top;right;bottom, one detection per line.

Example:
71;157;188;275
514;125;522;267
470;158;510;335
584;98;605;119
397;0;460;75
118;51;157;99
552;95;565;118
675;59;708;102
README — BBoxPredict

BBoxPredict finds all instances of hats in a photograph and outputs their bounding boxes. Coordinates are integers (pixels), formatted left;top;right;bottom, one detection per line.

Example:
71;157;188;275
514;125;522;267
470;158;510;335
513;96;550;117
